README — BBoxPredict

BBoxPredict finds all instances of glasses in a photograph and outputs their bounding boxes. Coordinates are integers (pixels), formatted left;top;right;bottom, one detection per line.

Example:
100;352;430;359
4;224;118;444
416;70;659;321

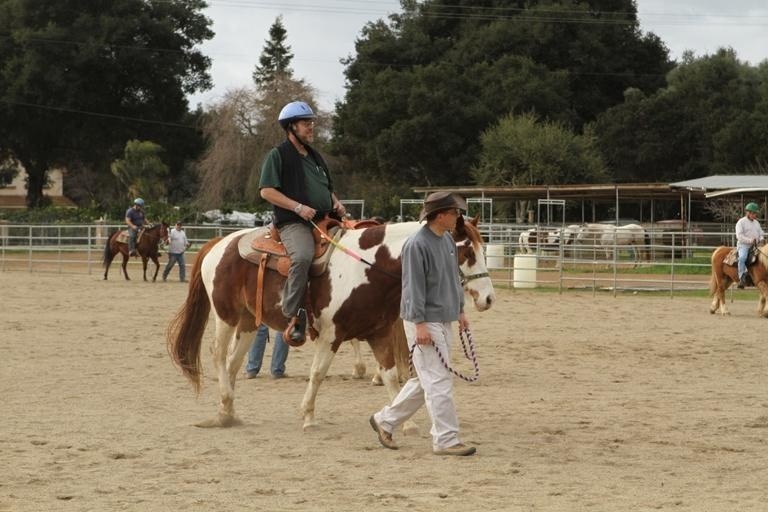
302;121;315;126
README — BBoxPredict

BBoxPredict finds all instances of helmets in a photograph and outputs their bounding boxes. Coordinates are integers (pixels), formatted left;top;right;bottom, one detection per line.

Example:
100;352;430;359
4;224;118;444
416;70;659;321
134;197;144;207
277;101;318;130
744;202;760;213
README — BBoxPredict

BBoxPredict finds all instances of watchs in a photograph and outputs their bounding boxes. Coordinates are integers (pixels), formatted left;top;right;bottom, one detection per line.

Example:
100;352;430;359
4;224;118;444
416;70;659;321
295;203;302;215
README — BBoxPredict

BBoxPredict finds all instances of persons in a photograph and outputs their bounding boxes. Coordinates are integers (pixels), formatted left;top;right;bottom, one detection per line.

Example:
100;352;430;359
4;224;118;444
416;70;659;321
125;197;149;257
369;191;477;457
162;221;190;283
258;101;347;343
246;323;289;379
735;202;765;289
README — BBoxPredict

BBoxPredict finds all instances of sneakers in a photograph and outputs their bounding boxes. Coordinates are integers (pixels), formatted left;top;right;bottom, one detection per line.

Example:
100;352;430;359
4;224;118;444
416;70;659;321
287;316;306;343
128;249;135;256
433;443;477;456
369;414;399;450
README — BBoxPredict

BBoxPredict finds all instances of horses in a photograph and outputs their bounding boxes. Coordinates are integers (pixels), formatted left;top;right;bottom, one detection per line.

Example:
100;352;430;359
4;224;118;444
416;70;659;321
709;243;768;317
103;221;169;282
168;211;497;435
518;224;581;255
576;222;651;269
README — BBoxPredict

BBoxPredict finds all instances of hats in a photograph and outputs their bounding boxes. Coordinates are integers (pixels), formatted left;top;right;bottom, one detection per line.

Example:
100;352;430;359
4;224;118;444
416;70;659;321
176;220;183;224
418;191;468;224
